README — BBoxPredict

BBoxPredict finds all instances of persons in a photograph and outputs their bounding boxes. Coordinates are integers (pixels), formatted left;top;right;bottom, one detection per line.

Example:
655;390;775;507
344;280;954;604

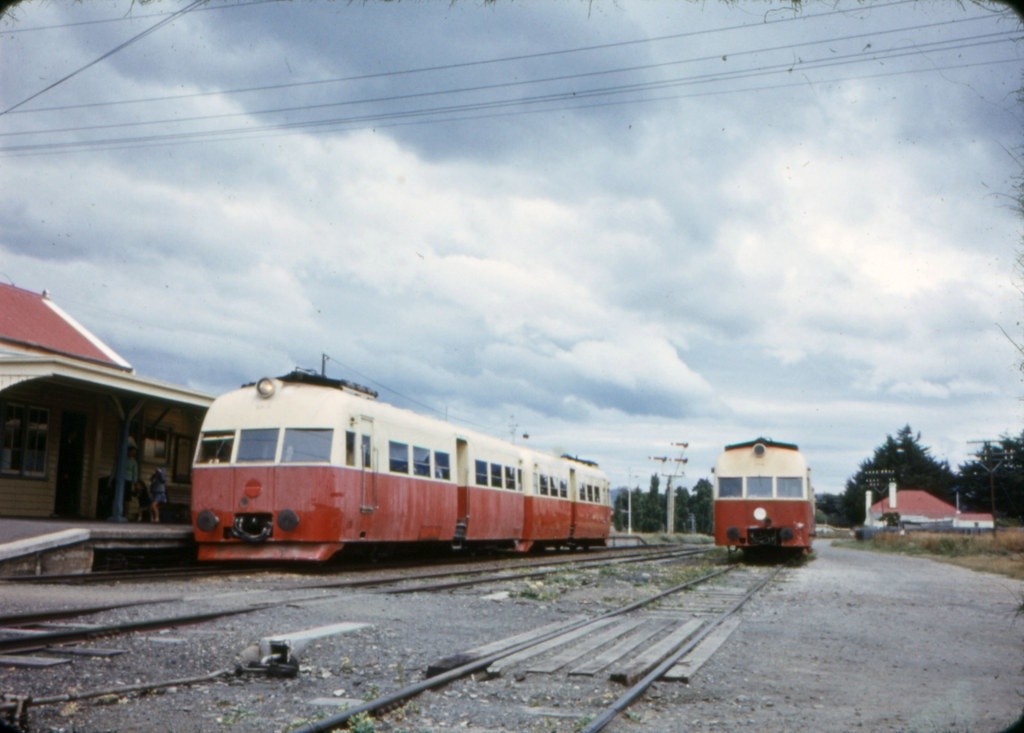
148;466;168;523
107;437;139;517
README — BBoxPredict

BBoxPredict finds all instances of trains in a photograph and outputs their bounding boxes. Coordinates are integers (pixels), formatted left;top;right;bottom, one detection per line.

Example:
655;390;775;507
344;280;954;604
190;371;614;571
710;435;817;567
